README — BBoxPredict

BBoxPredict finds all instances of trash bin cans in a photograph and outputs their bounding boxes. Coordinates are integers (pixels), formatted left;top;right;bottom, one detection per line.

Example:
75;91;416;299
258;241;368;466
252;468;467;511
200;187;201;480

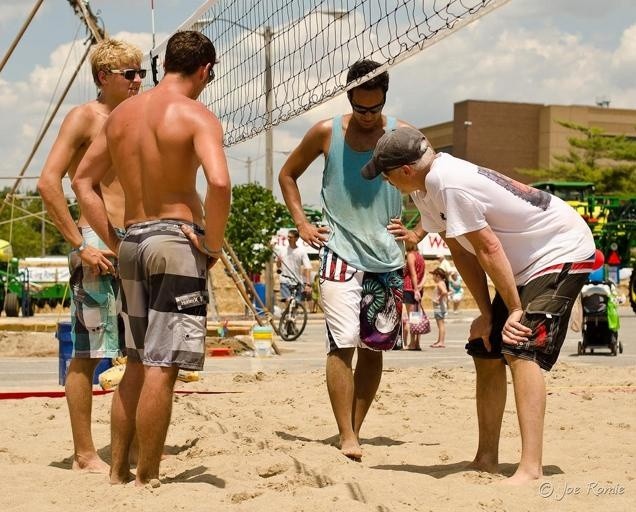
254;283;267;316
588;266;605;283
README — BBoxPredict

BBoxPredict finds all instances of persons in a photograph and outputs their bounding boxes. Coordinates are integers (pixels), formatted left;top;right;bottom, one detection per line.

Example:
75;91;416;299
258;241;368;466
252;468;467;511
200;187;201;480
67;25;235;494
36;34;148;478
276;57;435;463
356;125;601;485
429;254;464;350
273;229;314;322
400;240;427;352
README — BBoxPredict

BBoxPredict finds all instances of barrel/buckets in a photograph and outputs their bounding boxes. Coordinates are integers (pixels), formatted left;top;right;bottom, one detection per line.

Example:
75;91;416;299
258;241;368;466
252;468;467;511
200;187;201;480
253;326;274;356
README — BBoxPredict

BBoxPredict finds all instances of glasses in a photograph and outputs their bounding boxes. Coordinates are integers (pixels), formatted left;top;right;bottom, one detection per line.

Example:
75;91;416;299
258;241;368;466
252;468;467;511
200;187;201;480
208;67;215;83
380;162;416;177
105;68;147;81
350;100;384;114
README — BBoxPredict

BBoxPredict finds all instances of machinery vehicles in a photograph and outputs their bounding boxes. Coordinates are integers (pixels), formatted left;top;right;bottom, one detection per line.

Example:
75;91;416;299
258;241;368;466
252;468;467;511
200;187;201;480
0;257;36;317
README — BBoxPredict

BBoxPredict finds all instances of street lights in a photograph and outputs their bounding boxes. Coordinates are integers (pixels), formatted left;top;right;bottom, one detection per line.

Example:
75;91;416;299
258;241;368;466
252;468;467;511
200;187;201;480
190;7;354;314
218;145;295;184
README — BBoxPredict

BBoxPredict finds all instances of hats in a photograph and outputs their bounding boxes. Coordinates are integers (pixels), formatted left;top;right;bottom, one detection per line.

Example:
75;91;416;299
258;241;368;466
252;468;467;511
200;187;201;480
437;253;444;258
360;127;429;180
429;268;445;277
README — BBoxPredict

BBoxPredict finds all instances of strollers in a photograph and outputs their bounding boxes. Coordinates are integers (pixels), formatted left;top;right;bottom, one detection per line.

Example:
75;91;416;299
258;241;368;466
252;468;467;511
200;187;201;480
576;278;624;357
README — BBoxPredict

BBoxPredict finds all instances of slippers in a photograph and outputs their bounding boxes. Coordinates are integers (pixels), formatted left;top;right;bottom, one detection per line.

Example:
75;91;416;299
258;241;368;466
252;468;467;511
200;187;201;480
429;343;445;348
403;345;421;351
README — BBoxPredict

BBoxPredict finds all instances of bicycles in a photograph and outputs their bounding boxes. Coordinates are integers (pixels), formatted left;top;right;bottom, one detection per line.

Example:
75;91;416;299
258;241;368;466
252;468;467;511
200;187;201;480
278;272;311;343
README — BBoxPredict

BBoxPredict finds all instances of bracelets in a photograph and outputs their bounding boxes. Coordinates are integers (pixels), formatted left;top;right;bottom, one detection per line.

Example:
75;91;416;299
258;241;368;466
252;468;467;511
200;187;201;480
201;240;223;258
71;239;88;255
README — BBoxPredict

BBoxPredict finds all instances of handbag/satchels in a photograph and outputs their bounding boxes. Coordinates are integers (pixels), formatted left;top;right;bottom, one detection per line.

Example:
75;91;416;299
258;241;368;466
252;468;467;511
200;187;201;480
606;297;620;333
409;299;431;334
